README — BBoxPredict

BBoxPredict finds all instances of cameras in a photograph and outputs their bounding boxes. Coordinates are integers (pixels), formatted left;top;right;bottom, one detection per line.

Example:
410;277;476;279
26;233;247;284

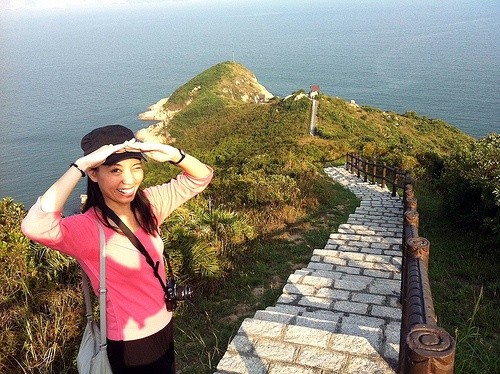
164;279;194;312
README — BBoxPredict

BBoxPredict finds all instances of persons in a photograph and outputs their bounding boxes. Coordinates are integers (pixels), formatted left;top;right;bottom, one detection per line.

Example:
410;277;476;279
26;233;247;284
21;125;215;374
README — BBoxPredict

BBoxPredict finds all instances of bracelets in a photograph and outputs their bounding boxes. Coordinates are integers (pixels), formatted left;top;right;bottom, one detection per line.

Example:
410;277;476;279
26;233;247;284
70;163;86;177
169;149;185;165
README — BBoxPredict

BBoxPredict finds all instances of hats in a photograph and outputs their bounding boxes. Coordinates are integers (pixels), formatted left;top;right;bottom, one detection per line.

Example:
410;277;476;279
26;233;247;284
81;125;148;165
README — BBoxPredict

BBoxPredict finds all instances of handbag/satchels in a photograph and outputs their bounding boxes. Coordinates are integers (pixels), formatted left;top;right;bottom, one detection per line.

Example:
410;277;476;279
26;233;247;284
77;320;112;374
